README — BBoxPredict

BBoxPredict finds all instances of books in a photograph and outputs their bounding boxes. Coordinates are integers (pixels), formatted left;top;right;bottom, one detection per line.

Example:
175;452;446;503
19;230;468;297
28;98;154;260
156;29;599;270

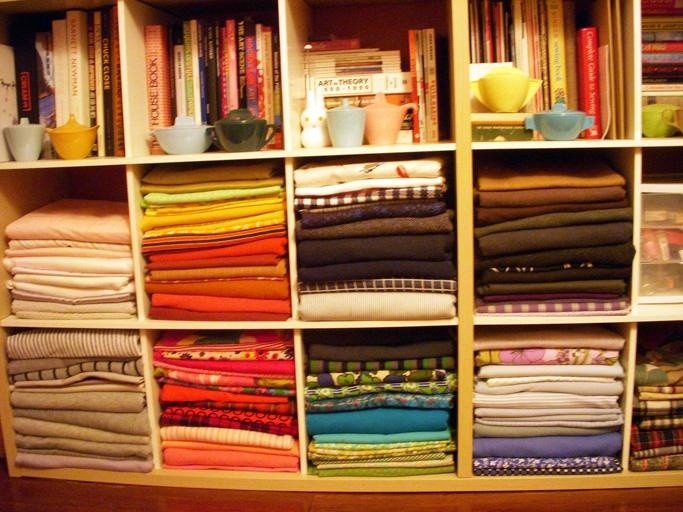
305;29;439;145
640;1;683;108
469;1;627;141
144;17;284;155
14;5;125;158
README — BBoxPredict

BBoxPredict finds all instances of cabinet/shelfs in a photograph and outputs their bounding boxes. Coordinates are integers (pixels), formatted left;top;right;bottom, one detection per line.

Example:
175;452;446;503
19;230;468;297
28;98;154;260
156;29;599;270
0;0;683;493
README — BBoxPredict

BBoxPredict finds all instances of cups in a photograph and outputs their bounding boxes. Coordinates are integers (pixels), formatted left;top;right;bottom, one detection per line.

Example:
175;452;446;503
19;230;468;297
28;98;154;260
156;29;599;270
45;114;100;159
214;108;276;152
364;91;419;146
153;115;213;154
524;102;596;140
3;117;46;161
470;68;543;113
326;97;365;147
641;103;682;137
